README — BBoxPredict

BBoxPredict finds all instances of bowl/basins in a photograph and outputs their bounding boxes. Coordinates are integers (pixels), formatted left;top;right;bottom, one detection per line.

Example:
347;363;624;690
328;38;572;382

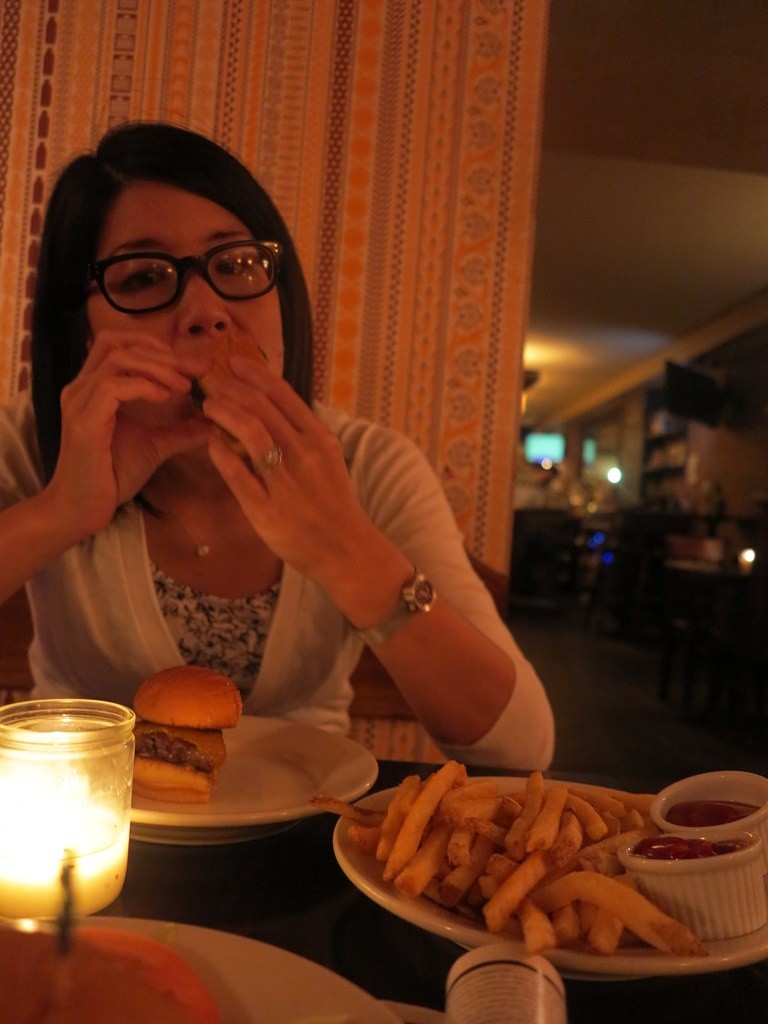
618;831;768;942
651;770;768;857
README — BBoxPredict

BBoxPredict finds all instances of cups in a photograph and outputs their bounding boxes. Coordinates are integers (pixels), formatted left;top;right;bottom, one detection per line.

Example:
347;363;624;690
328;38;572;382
0;697;135;921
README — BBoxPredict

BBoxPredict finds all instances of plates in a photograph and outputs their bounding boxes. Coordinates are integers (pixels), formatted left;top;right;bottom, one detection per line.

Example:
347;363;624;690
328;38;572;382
82;916;403;1024
332;777;768;982
127;715;379;844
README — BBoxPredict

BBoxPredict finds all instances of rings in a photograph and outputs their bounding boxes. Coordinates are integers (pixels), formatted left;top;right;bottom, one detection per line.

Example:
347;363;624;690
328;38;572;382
254;448;282;477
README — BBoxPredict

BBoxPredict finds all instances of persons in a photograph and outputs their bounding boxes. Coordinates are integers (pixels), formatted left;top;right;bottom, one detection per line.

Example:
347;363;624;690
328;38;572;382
1;122;555;773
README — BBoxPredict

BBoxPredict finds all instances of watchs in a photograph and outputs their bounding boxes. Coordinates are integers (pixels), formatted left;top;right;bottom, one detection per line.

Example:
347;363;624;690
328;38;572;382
352;566;437;644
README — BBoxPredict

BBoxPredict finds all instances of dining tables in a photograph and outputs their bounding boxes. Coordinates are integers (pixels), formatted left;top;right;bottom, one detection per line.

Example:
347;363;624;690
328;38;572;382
80;759;768;1024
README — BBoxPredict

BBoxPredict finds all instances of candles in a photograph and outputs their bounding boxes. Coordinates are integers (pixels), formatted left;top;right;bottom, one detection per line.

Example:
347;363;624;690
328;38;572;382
0;737;93;886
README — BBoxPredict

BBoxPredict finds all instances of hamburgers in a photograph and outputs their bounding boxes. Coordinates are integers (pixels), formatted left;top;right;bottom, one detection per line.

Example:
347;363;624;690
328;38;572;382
0;856;223;1024
125;664;243;805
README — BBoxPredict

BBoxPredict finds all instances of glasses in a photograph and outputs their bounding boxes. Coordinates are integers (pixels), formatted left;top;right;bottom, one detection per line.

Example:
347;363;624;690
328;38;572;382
93;241;284;316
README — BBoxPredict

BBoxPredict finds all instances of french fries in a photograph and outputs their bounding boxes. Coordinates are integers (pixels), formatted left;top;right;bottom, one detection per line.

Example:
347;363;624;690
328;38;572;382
309;761;711;959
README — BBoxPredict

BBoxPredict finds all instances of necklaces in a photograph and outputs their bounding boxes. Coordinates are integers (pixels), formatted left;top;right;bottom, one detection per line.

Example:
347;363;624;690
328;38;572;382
158;487;232;556
